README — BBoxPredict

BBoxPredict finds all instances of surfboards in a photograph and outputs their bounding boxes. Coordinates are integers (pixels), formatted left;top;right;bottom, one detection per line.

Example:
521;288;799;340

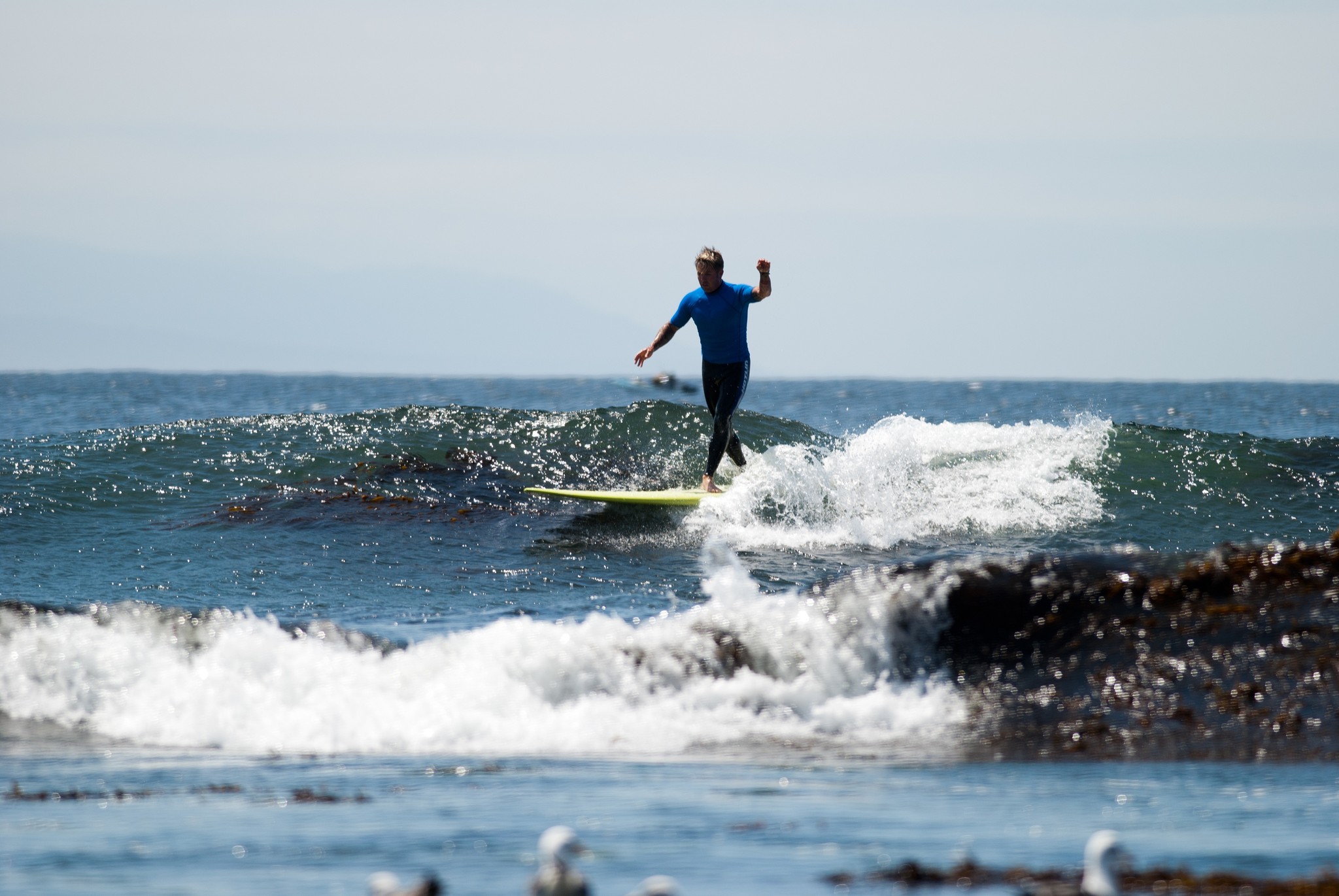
523;487;731;506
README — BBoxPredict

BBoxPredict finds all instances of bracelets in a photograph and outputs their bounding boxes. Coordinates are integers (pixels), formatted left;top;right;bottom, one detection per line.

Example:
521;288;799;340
760;271;770;274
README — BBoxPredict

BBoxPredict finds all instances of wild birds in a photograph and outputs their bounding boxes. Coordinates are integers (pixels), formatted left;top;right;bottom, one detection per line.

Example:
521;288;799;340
1074;826;1140;896
622;872;687;896
525;822;594;896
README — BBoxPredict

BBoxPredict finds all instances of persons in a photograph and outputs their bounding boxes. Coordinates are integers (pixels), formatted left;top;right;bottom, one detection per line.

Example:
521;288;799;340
634;246;772;492
